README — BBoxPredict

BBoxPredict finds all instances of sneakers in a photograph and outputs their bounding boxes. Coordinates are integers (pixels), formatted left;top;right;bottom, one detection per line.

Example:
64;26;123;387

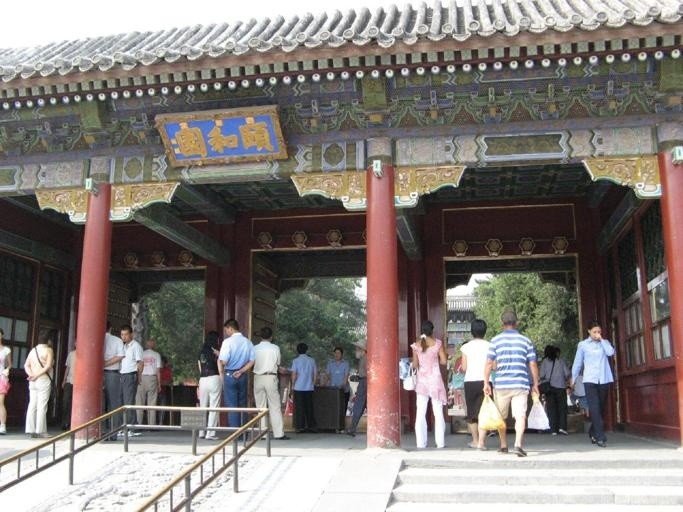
205;435;219;440
514;447;527;457
0;426;6;434
559;429;568;434
496;448;508;453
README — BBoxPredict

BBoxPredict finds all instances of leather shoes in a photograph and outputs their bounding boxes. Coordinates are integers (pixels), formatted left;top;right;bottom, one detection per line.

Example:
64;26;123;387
275;435;289;440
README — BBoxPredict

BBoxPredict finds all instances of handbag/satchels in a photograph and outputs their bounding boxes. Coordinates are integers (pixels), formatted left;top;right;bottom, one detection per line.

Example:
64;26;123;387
539;382;550;394
403;365;417;391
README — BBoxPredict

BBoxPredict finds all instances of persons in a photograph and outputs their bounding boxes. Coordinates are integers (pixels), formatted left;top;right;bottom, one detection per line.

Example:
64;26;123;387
101;320;126;441
213;319;256;441
198;330;222;440
285;339;367;438
460;320;497;451
410;321;447;448
252;328;291;440
0;325;173;437
455;341;590;437
567;321;615;447
23;329;54;438
482;310;540;458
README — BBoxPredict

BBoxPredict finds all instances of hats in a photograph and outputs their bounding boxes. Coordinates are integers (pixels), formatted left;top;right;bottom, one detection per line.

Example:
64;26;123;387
352;339;367;351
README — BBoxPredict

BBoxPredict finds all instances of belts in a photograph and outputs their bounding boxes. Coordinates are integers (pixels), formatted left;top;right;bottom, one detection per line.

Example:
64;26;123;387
264;373;276;375
